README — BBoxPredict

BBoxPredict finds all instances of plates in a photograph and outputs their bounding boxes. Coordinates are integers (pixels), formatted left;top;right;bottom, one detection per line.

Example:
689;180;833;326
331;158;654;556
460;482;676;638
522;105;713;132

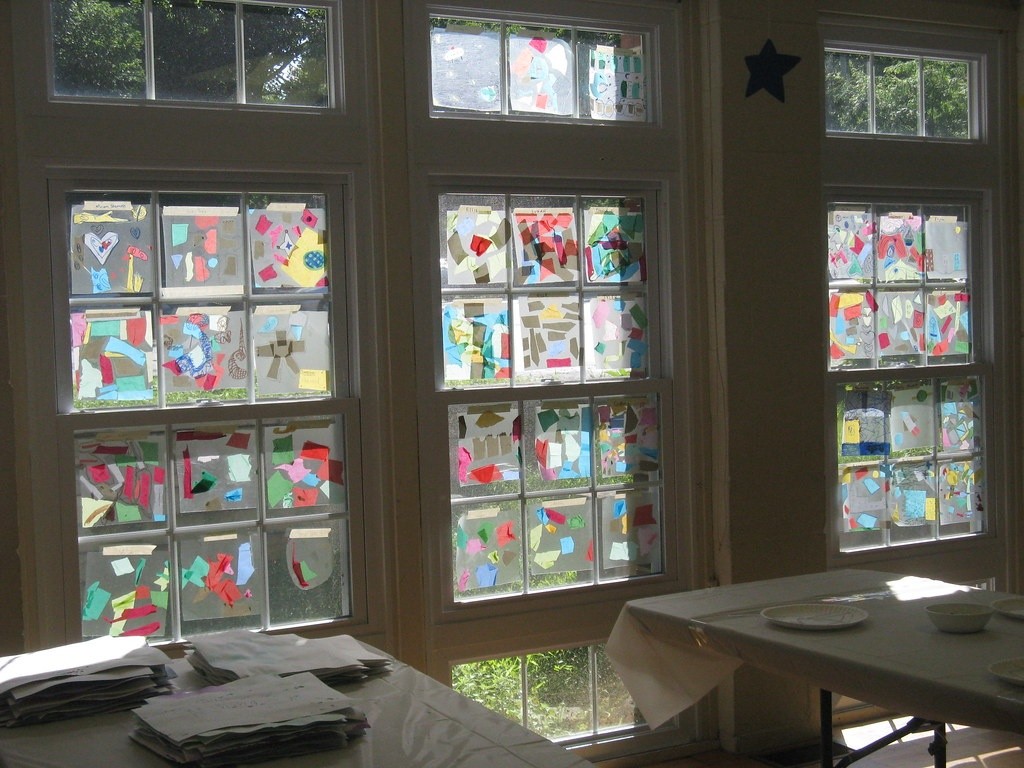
987;655;1024;684
761;603;872;633
990;597;1024;618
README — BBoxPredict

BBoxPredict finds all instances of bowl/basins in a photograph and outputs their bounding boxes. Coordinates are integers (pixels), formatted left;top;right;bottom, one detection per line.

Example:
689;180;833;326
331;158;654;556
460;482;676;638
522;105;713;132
925;603;992;635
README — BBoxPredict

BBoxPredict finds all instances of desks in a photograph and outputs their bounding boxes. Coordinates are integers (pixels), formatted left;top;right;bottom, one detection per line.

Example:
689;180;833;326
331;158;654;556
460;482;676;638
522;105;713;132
604;568;1024;768
0;634;596;768
